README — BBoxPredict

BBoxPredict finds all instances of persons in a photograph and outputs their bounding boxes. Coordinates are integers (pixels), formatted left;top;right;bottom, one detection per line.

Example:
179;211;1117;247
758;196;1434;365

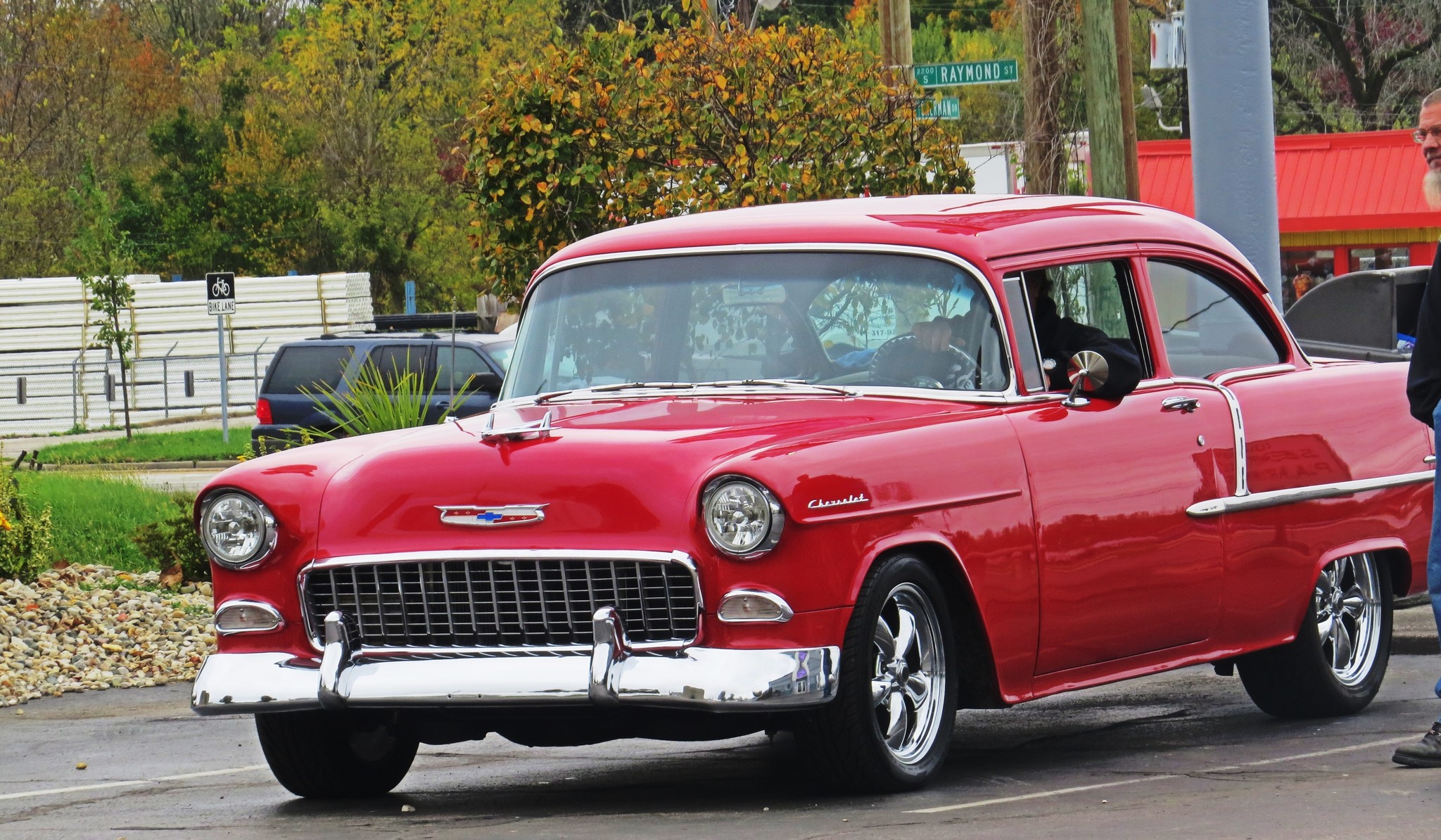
899;266;1146;396
1287;89;1441;769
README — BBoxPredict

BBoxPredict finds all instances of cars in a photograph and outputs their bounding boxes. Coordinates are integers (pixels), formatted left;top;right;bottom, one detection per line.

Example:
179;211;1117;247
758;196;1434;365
188;193;1439;802
1280;264;1433;363
251;331;515;458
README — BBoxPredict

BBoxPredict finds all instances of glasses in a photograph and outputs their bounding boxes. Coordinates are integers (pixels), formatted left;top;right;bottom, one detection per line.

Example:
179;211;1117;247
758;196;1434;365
1411;126;1441;143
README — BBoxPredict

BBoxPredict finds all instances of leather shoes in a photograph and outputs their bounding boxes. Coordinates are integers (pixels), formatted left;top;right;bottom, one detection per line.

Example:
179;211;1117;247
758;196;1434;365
1391;721;1441;768
